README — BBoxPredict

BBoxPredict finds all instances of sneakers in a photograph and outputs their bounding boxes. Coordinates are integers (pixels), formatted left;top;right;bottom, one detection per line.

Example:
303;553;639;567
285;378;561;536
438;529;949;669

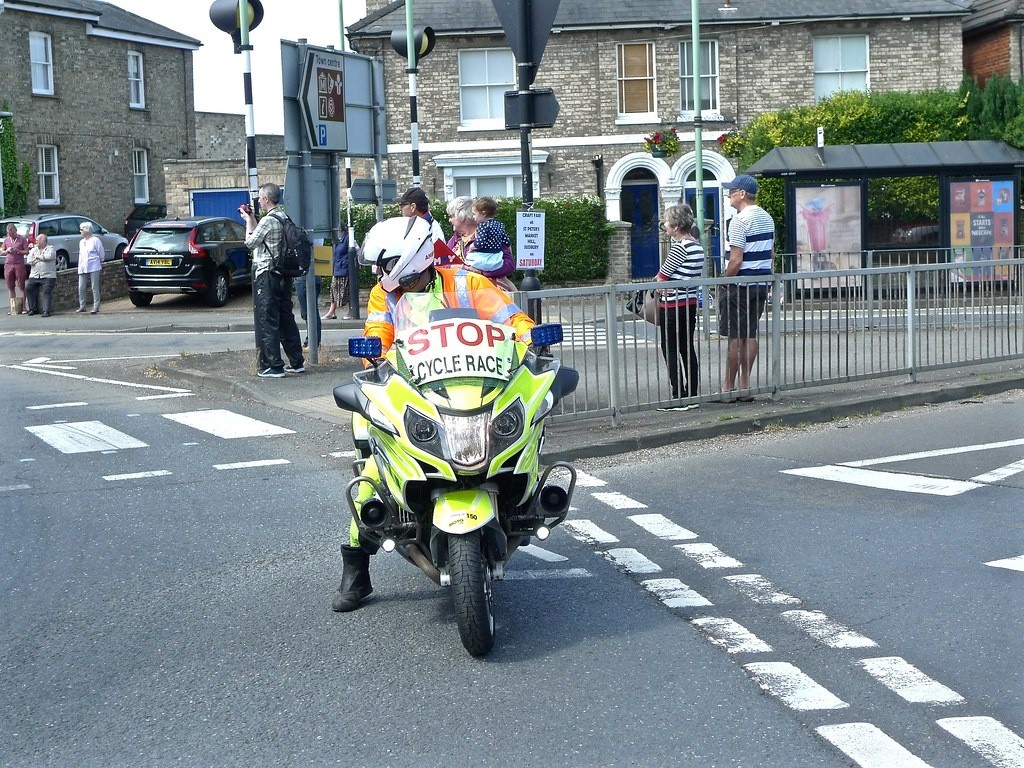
284;364;305;373
257;368;286;378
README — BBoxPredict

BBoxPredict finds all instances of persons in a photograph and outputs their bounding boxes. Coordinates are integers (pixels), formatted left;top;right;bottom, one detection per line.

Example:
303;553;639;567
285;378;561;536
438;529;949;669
293;239;323;349
25;234;57;317
330;216;552;612
393;188;445;244
321;221;361;320
75;222;105;313
653;203;705;412
446;196;519;294
2;223;29;315
240;184;306;378
709;174;775;404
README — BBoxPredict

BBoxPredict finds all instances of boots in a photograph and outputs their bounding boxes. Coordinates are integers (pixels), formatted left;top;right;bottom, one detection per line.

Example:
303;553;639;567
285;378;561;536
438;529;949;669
332;544;373;613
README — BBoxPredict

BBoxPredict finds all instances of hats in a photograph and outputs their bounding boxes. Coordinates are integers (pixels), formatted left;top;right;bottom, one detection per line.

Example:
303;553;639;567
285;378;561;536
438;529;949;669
401;187;429;209
722;174;758;194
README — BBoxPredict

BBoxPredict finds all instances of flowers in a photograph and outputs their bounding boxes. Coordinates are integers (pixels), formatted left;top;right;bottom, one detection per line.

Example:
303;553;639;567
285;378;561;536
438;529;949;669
644;126;682;158
717;132;744;157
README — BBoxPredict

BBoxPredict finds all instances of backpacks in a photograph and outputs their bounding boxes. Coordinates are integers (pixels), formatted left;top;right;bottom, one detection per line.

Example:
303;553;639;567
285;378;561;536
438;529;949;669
263;213;311;278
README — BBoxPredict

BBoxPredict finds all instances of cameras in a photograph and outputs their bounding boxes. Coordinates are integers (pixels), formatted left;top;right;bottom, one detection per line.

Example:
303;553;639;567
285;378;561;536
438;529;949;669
238;204;252;215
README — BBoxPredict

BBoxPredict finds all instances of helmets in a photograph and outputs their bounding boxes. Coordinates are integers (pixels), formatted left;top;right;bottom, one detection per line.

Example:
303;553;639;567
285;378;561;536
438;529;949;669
357;214;434;294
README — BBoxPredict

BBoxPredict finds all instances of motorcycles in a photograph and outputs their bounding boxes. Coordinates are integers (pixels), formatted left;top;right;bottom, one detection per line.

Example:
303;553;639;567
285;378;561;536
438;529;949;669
333;288;576;659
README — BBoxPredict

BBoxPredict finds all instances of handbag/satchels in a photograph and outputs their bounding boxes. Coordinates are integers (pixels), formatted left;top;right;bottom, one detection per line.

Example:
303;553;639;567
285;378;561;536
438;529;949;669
354;240;360;251
625;276;662;326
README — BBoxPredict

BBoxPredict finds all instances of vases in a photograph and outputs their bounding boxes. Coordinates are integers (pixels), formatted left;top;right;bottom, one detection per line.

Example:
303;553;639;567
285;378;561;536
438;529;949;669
650;143;667;158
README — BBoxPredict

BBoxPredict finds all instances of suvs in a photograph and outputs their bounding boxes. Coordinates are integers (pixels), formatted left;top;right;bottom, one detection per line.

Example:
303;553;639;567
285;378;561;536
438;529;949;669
121;217;251;307
0;213;130;272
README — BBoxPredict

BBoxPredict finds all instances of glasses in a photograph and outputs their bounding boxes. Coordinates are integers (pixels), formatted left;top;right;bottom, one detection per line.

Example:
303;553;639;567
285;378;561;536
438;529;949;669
729;189;740;195
399;203;408;208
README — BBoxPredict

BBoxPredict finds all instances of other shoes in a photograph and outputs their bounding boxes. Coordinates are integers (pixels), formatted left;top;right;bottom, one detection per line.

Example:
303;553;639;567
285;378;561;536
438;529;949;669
342;315;352;319
27;310;37;316
740;397;754;401
22;310;27;314
76;307;87;313
42;311;50;316
302;345;310;353
320;315;337;320
90;308;99;313
713;389;737;403
8;311;11;315
657;405;689;411
689;403;700;409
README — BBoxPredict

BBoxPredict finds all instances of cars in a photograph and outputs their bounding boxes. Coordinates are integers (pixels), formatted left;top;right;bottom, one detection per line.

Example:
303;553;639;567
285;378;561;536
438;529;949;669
124;203;168;241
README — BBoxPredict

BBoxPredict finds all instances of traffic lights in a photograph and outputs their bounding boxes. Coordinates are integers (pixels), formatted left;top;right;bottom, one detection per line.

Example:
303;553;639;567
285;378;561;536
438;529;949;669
391;26;436;58
210;0;265;34
